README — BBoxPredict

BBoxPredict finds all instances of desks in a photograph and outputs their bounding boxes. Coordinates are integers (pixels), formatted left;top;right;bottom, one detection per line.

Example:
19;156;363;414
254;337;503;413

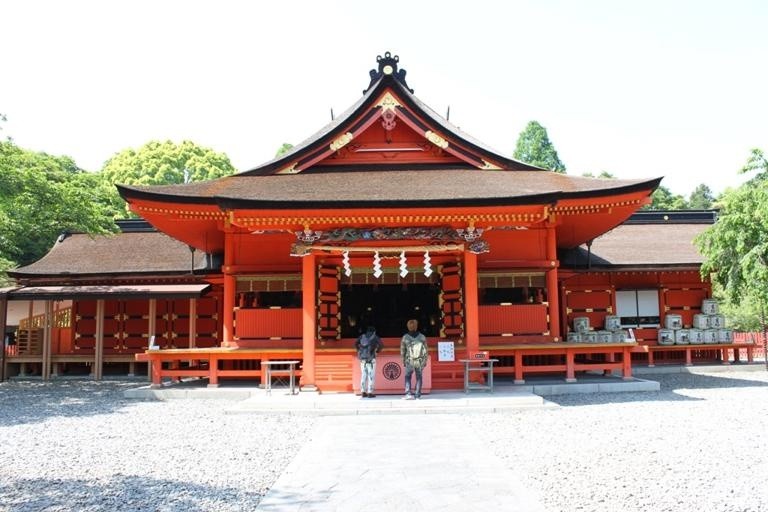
457;357;500;395
260;360;301;395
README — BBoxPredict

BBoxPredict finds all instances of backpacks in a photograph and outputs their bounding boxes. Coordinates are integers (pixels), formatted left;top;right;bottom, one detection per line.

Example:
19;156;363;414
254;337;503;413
406;336;428;369
358;334;376;361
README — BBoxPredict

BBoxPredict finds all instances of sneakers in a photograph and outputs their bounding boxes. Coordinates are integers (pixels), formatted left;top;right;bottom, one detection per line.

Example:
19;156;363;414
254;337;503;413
402;395;423;402
361;391;376;400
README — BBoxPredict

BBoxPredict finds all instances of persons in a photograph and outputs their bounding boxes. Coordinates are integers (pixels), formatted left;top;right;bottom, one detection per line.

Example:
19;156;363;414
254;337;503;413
354;325;386;399
400;318;430;401
